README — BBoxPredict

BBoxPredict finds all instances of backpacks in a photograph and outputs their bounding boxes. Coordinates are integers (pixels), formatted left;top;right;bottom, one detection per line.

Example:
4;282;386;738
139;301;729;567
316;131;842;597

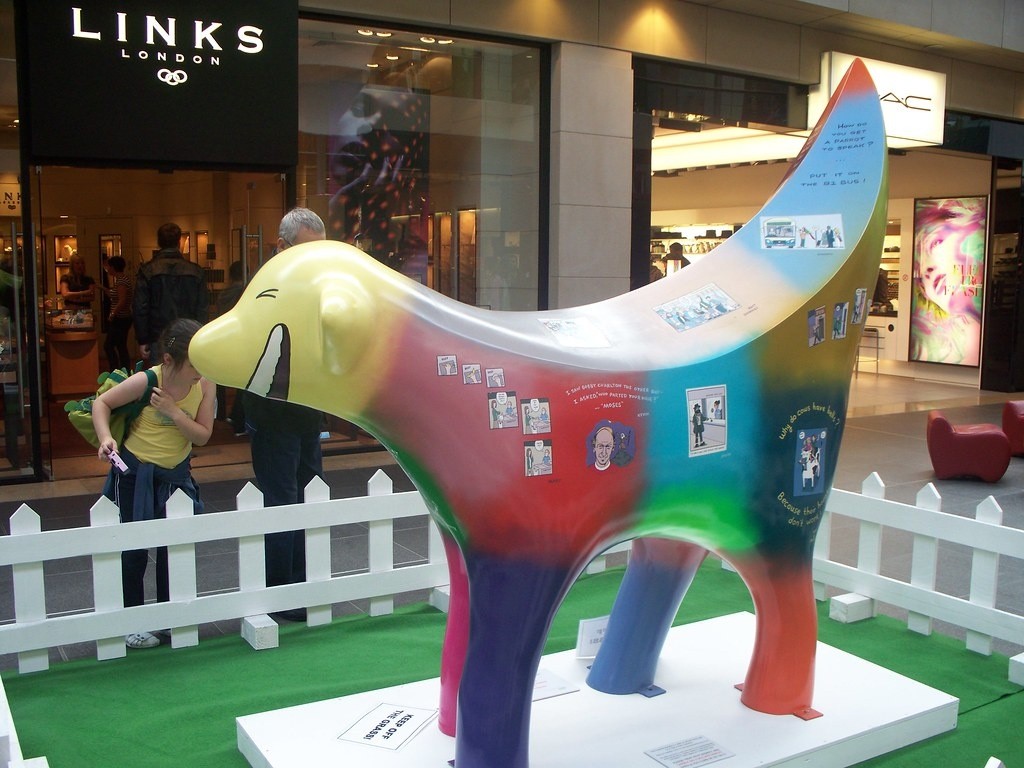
64;360;158;451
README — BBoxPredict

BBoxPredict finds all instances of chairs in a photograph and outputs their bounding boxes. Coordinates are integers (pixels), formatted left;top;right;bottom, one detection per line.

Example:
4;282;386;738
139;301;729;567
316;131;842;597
926;411;1010;483
1001;400;1024;457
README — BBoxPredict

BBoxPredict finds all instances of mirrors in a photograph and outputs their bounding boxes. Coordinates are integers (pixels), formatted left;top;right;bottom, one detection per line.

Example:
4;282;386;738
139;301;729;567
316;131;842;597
99;233;123;333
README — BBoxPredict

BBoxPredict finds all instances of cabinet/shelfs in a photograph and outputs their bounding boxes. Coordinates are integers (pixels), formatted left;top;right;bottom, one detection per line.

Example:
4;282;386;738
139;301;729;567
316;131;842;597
878;251;899;282
54;234;77;294
650;239;728;259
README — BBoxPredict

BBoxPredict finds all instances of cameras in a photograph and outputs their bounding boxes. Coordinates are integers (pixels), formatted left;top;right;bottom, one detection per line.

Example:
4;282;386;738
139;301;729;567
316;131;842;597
108;451;130;476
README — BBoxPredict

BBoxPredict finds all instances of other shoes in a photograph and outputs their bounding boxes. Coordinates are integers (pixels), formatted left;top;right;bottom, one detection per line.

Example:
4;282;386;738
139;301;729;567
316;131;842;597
234;429;249;436
125;632;160;648
226;417;232;423
159;629;171;636
271;608;307;622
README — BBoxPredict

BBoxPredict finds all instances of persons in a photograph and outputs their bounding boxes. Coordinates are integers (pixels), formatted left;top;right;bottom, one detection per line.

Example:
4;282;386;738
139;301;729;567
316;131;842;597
92;316;216;649
95;256;134;377
240;209;330;621
218;260;251;437
60;254;96;310
915;207;984;316
134;223;209;366
650;242;690;283
